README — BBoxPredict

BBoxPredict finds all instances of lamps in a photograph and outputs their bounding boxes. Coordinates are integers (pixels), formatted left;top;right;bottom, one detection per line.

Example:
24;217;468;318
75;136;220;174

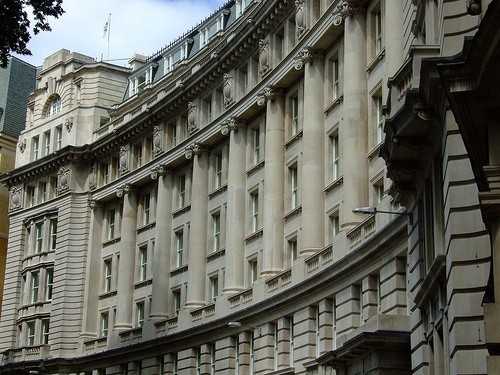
29;370;45;375
351;207;413;226
228;321;261;337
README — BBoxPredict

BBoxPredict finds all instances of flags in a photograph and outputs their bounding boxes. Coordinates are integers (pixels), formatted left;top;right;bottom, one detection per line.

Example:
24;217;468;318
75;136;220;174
103;17;109;37
